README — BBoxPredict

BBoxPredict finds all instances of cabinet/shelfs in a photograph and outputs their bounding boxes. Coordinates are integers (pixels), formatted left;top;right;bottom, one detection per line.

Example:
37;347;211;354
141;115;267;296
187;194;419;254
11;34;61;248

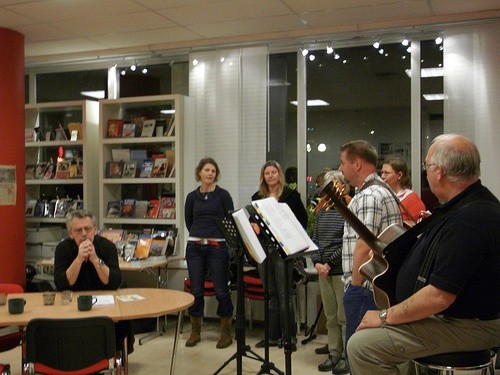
25;99;98;261
99;94;193;263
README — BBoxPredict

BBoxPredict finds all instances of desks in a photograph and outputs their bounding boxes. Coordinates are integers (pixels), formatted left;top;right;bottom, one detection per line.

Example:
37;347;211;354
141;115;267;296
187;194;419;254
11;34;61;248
0;288;195;375
37;255;184;346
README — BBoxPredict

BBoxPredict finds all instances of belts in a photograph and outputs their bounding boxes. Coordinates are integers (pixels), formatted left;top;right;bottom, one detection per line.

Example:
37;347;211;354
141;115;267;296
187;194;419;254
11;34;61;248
196;239;220;249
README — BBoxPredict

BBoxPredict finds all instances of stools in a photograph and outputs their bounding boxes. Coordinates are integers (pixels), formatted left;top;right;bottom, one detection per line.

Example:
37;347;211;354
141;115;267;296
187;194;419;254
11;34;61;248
412;348;497;375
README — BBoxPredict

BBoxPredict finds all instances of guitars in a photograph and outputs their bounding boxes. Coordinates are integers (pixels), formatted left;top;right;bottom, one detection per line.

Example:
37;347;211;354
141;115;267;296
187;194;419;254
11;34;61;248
314;176;419;309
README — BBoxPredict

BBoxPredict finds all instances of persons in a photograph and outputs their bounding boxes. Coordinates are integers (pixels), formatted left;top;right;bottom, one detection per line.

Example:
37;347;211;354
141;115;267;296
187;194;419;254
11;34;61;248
310;171;358;374
53;210;121;291
380;157;429;234
347;135;499;375
250;160;308;353
184;157;234;348
283;160;439;216
339;140;404;374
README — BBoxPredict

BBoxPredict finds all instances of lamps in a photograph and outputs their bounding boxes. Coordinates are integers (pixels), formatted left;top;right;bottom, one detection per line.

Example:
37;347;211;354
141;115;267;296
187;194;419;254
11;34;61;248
299;47;308;56
326;42;333;54
434;32;442;44
121;67;130;75
402;33;409;46
373;38;383;49
131;59;138;71
141;65;150;74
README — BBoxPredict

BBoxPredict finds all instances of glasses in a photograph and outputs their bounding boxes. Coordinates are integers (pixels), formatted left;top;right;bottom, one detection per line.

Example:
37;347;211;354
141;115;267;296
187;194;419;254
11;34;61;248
381;171;397;175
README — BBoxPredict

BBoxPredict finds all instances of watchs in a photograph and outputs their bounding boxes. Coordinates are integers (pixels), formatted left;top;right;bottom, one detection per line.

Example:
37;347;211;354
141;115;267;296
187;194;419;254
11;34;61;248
380;307;390;328
96;259;104;269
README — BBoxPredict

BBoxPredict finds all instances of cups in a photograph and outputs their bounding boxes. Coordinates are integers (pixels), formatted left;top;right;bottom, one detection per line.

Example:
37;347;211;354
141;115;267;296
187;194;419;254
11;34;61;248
61;290;72;303
9;298;26;313
0;292;7;305
77;295;97;310
42;294;56;305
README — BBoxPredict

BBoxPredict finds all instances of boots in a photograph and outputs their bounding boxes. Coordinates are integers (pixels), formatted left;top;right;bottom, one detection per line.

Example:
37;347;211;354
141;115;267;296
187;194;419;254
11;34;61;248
186;316;202;346
216;319;233;348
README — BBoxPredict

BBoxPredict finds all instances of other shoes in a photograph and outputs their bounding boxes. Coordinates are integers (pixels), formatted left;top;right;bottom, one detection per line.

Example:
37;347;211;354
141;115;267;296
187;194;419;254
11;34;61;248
318;357;339;371
315;344;329;354
283;342;297;352
255;340;278;348
333;358;350;374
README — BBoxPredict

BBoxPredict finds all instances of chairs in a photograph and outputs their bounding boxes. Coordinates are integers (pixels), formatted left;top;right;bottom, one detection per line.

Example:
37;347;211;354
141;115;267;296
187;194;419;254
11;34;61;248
184;274;302;336
18;316;121;375
